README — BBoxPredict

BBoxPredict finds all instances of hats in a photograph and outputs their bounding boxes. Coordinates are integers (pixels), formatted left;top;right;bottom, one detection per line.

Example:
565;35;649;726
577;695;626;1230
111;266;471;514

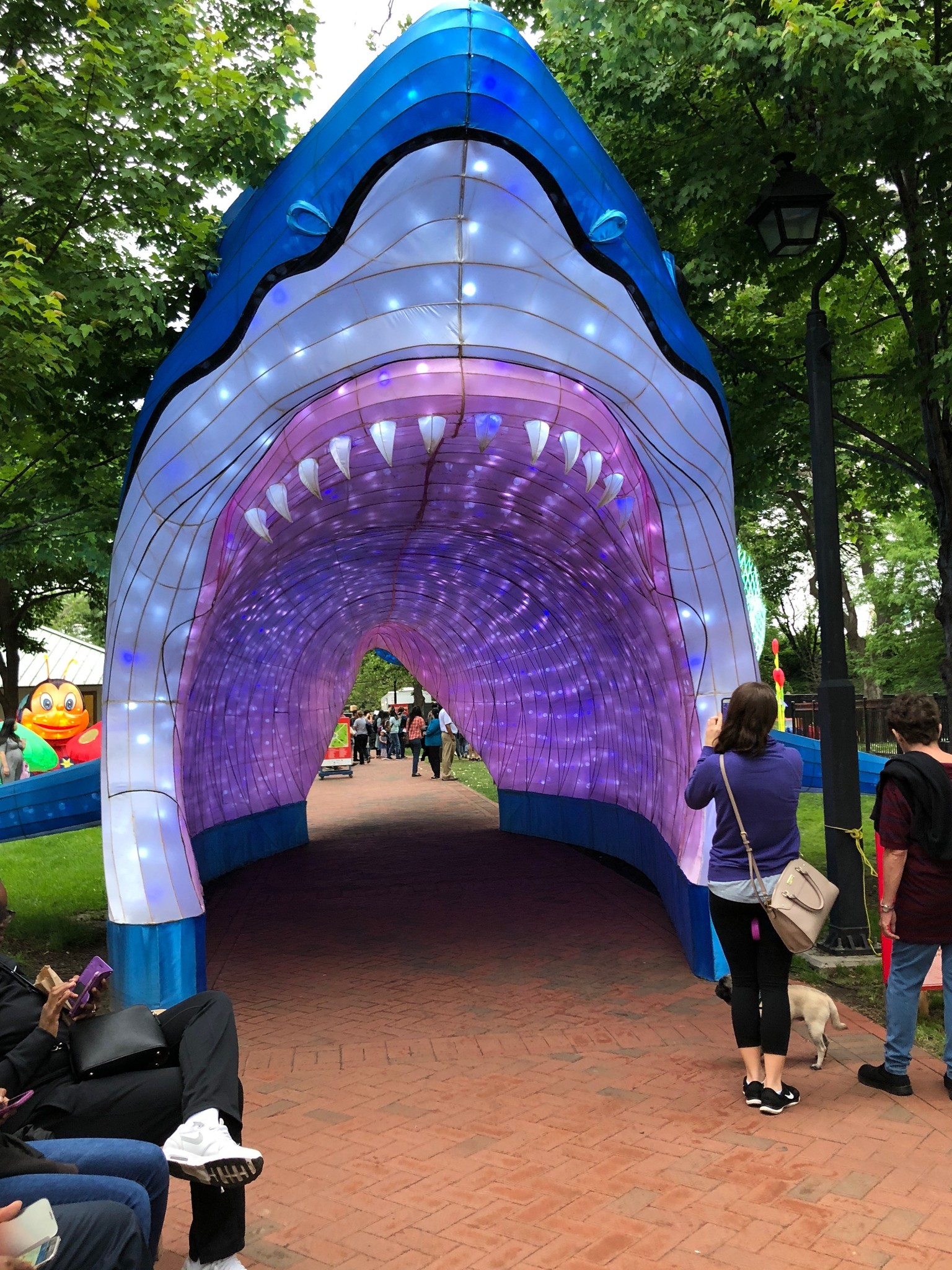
359;710;364;714
382;729;386;733
432;708;439;715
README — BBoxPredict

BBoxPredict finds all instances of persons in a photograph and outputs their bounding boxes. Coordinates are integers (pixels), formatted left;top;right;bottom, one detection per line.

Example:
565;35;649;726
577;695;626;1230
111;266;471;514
857;690;952;1103
0;876;265;1270
683;681;804;1116
0;718;24;785
318;705;483;781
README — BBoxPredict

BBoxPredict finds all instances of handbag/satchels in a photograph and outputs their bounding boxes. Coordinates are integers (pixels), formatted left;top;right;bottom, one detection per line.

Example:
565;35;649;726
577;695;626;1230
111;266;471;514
77;1003;167;1080
766;859;839;955
365;719;372;736
404;733;409;748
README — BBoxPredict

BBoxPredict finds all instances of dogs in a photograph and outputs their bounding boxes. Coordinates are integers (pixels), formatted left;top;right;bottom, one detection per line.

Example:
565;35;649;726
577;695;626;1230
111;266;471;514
715;973;849;1071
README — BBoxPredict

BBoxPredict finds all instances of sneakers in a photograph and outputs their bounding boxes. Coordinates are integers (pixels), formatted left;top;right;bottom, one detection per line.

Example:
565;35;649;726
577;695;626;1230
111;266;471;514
759;1081;801;1114
162;1117;264;1188
182;1254;247;1270
858;1061;914;1096
742;1076;763;1105
943;1072;952;1100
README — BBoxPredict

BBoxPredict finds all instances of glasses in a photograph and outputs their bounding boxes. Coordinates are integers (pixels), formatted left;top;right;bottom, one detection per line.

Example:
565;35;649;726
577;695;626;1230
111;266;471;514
0;908;16;929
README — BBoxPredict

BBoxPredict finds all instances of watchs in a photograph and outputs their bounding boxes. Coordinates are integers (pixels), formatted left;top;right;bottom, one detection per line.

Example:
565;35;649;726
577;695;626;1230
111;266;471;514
880;898;896;912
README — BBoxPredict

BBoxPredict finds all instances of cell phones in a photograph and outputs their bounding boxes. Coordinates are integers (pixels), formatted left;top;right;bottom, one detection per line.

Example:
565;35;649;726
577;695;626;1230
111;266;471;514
721;697;732;723
0;1090;34;1114
69;955;113;1016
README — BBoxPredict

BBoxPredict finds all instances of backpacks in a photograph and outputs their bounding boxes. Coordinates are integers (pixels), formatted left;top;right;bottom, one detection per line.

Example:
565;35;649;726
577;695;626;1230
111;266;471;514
384;720;394;732
377;725;383;735
372;718;379;733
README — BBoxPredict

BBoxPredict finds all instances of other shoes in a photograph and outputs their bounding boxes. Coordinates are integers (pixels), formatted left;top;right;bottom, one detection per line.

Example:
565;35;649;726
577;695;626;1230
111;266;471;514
430;775;440;780
325;767;334;770
351;762;359;767
442;775;458;781
411;773;421;777
353;756;408;764
334;766;343;770
458;755;480;762
